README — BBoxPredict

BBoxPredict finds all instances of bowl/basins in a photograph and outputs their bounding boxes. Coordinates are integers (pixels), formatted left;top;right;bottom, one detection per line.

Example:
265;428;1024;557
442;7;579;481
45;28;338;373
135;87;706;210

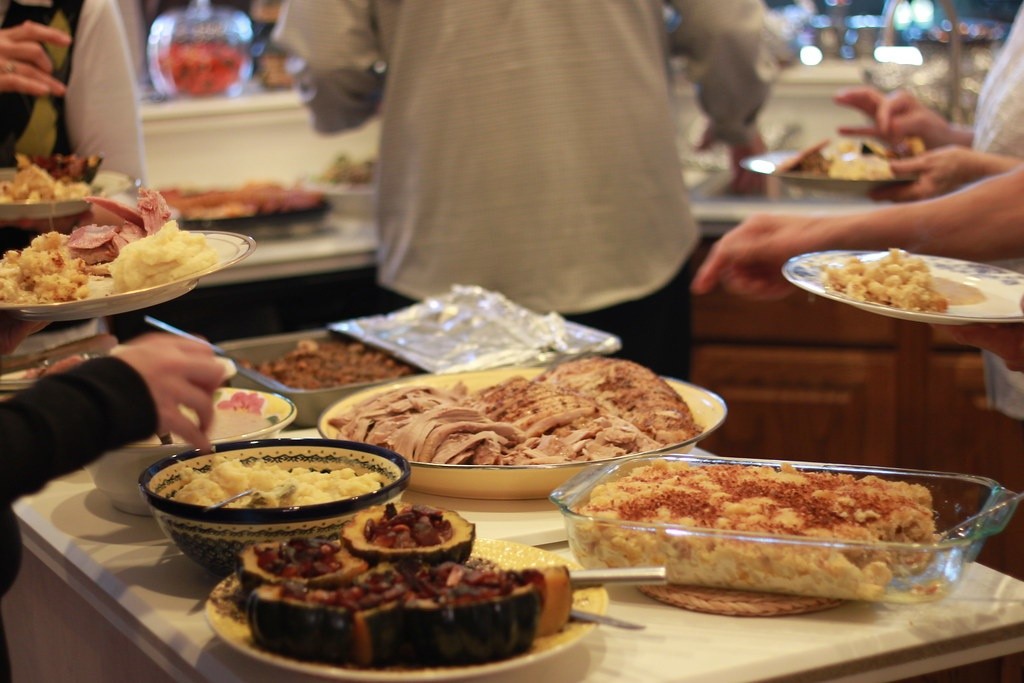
85;387;299;518
136;438;413;577
323;188;376;216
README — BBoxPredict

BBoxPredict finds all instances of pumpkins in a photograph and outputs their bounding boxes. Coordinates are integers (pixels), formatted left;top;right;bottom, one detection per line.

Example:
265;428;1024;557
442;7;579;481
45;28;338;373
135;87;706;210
232;501;575;672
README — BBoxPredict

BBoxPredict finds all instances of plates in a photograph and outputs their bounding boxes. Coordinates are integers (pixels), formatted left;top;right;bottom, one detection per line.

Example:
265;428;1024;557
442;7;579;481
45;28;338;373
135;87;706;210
781;249;1024;325
0;167;144;219
0;229;257;323
205;537;609;681
164;184;332;241
738;148;918;194
318;366;729;501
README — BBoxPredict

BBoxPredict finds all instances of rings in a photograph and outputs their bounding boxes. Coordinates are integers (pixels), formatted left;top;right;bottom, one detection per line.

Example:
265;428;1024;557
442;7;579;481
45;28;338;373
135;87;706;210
3;61;23;74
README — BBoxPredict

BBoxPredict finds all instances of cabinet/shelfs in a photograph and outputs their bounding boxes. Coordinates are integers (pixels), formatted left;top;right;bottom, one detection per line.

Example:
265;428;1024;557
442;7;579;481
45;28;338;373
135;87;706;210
689;241;1024;683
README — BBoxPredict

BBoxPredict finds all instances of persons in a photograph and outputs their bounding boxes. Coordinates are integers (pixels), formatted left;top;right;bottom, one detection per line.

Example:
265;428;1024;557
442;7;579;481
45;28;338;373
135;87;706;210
273;1;797;385
693;0;1024;585
0;319;224;683
0;0;144;188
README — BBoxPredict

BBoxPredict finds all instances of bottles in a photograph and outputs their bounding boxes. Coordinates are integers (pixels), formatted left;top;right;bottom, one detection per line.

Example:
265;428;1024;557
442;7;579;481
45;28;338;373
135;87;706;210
147;1;255;98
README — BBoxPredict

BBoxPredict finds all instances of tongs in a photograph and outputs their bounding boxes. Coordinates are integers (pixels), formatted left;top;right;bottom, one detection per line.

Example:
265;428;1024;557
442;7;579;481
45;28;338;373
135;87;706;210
568;566;677;633
